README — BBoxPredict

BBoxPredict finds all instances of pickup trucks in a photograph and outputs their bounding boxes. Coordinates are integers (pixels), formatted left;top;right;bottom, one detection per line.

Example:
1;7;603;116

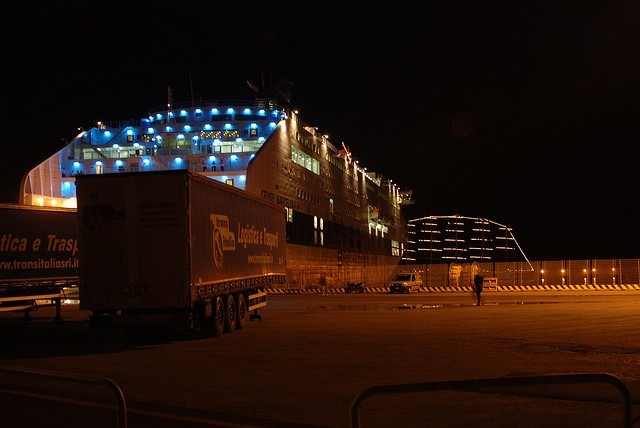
345;281;364;293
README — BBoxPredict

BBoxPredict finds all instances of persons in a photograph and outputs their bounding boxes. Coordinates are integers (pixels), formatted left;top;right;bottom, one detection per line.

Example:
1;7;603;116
211;161;216;171
62;168;66;177
60;167;64;174
71;170;82;176
219;159;225;171
474;274;483;306
200;160;207;172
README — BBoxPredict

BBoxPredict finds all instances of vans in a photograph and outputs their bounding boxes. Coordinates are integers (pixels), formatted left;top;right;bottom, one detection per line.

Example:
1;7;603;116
389;273;422;294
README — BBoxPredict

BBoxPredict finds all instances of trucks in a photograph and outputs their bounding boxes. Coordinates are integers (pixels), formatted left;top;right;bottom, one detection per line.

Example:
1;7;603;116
76;169;287;338
